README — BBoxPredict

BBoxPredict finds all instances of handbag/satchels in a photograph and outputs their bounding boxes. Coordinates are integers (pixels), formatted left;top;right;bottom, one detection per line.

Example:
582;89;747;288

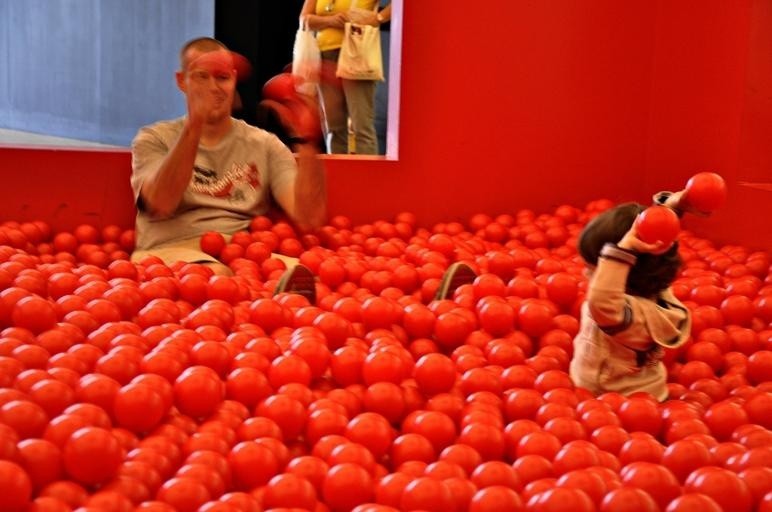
336;22;386;82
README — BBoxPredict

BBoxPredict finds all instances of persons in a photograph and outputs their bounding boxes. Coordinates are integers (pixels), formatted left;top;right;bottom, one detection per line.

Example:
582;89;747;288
298;0;392;155
129;37;477;302
571;191;692;402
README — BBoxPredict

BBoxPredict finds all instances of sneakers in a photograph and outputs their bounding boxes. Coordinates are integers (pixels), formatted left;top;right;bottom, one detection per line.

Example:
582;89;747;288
272;264;316;306
435;261;477;300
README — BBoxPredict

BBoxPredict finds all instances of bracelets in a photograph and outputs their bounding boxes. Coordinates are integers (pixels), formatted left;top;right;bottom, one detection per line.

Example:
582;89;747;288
377;12;386;24
290;136;310;144
